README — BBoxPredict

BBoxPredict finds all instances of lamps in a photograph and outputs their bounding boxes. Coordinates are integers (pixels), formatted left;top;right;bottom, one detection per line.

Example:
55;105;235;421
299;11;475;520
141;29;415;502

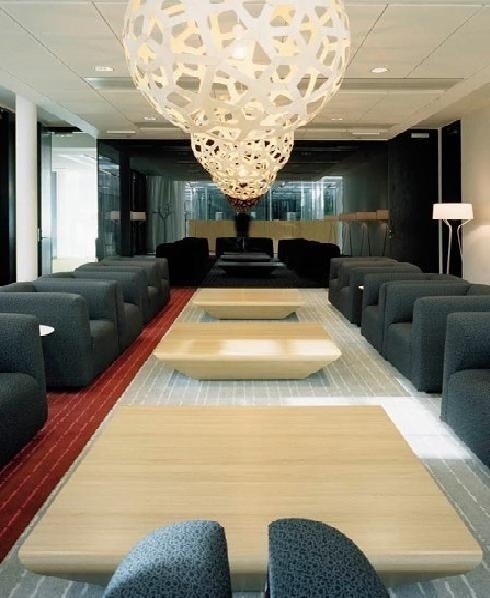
129;212;147;256
104;211;120;254
211;176;277;200
123;0;351;140
432;204;474;274
188;141;296;178
339;209;389;257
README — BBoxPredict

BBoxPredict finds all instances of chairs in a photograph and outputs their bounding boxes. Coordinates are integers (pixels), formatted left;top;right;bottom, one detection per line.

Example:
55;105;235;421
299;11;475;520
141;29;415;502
265;518;390;598
156;237;340;288
103;520;232;598
328;257;490;468
0;257;171;466
